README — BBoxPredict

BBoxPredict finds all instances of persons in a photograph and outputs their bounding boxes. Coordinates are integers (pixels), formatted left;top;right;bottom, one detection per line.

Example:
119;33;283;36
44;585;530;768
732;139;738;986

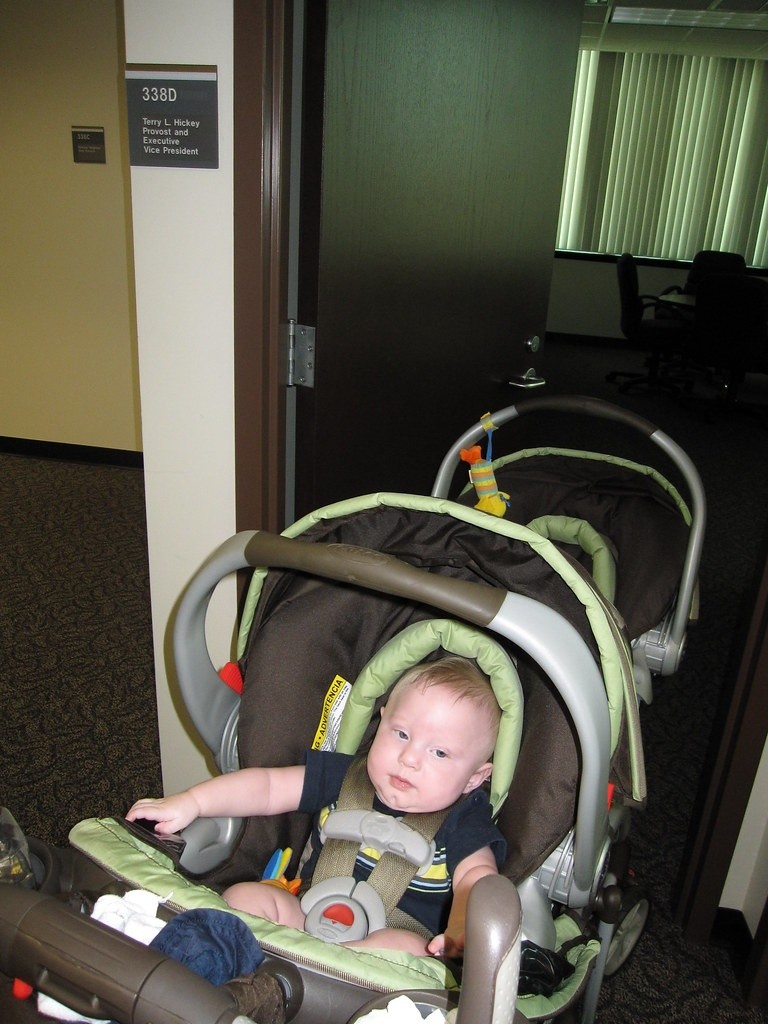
124;654;516;960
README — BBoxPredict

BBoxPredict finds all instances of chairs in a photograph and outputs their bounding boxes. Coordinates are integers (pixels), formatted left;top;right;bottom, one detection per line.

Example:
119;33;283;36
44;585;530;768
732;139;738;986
677;272;768;430
605;252;697;393
686;250;746;387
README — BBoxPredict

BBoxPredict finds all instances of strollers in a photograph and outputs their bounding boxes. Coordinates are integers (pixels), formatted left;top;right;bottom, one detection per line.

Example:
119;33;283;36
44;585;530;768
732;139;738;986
1;395;707;1023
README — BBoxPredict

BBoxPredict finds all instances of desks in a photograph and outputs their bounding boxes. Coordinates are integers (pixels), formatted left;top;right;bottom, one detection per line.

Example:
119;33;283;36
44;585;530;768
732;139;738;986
659;295;696;314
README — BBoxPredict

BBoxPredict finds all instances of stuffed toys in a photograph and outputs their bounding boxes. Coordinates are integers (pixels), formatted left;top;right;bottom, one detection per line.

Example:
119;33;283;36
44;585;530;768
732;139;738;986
458;429;511;518
